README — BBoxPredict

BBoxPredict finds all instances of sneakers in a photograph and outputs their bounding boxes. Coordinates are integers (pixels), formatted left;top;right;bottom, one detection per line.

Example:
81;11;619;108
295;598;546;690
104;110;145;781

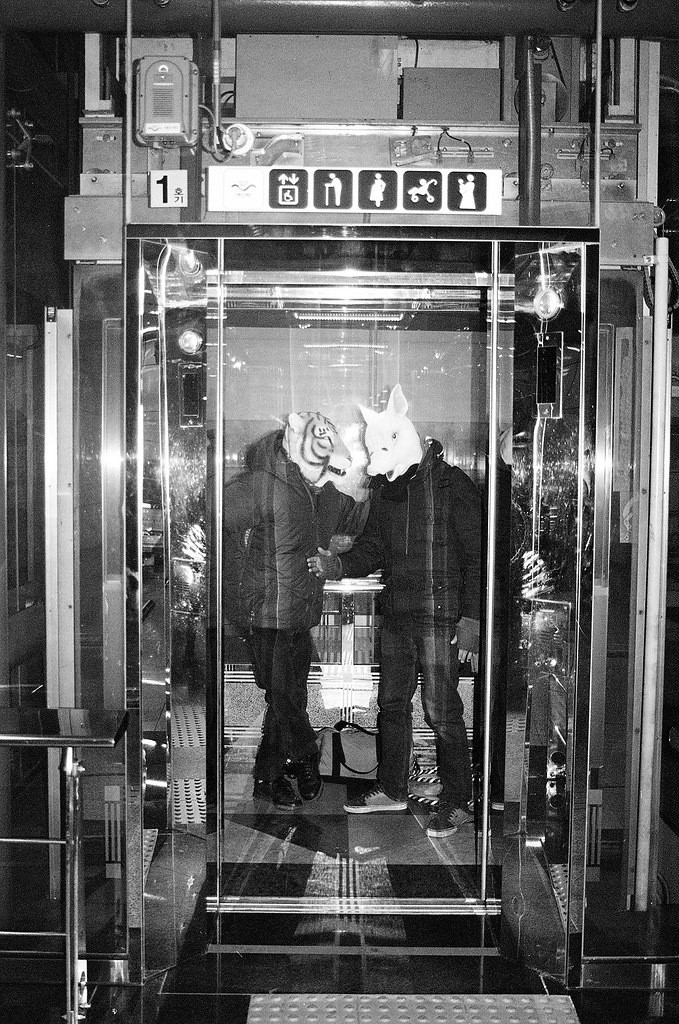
343;784;408;813
426;797;475;837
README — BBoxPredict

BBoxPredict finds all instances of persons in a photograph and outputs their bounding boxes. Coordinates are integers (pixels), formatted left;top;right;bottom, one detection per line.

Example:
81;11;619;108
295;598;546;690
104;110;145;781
220;412;374;811
306;384;489;838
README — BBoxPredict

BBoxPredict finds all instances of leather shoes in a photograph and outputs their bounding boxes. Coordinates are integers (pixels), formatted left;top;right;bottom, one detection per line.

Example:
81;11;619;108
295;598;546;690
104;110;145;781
297;751;325;803
252;778;303;811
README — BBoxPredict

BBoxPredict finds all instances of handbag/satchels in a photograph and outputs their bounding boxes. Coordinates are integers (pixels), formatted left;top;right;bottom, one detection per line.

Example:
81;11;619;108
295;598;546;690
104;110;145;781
314;720;420;783
